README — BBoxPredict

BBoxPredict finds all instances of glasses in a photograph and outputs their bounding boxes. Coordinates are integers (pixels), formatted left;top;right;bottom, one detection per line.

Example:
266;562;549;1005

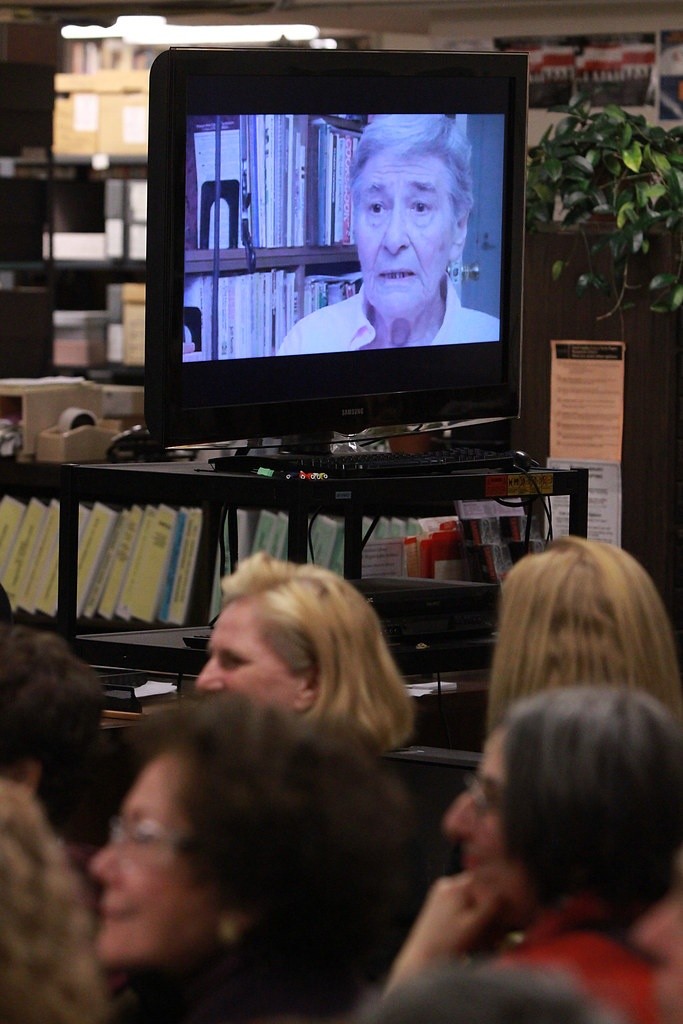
460;765;513;806
109;813;217;874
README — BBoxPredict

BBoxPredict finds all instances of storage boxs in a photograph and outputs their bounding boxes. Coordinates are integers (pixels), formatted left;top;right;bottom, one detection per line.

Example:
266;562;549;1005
52;68;150;163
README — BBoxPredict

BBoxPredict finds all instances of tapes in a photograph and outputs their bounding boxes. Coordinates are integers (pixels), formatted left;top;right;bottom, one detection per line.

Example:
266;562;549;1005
57;408;98;433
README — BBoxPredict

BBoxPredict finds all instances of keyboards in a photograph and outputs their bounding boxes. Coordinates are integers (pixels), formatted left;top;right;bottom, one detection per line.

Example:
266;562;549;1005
208;446;513;478
183;634;211;649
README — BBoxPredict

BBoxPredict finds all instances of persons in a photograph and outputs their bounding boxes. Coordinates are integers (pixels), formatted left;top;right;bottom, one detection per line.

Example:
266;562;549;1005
276;113;500;356
0;539;683;1024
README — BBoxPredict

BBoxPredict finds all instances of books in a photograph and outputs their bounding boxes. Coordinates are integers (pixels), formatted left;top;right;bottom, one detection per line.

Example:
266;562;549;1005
185;113;364;362
0;494;425;629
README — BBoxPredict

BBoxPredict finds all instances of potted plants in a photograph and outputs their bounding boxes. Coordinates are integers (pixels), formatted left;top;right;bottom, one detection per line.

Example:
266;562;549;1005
524;91;683;324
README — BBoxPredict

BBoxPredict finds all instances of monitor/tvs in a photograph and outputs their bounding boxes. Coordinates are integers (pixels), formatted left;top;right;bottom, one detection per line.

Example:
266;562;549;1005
140;49;530;449
